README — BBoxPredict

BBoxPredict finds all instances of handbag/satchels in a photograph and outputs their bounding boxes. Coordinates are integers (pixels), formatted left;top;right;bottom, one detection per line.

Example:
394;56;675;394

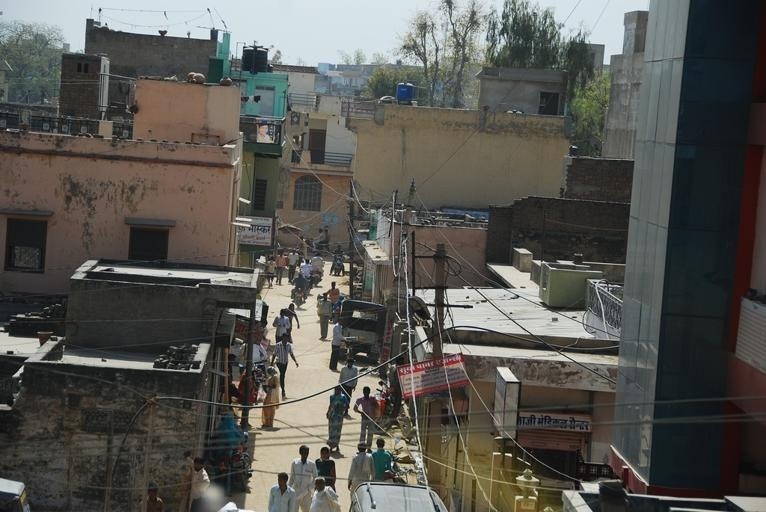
328;499;341;512
298;490;312;512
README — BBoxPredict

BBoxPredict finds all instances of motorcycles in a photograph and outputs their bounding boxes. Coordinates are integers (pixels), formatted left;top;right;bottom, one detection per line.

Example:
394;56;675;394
291;262;325;308
372;381;395;435
317;294;344;317
331;250;345;277
218;432;251;492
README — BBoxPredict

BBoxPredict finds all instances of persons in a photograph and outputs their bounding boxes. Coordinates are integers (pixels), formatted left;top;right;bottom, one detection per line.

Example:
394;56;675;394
192;457;210;504
339;358;358;416
317;281;343;371
139;482;166;512
256;124;272;142
354;386;378;452
268;438;393;512
226;304;300;427
326;387;347;452
265;228;346;304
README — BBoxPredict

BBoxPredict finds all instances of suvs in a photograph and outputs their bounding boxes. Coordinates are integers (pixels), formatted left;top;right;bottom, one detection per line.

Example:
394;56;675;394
338;299;387;364
